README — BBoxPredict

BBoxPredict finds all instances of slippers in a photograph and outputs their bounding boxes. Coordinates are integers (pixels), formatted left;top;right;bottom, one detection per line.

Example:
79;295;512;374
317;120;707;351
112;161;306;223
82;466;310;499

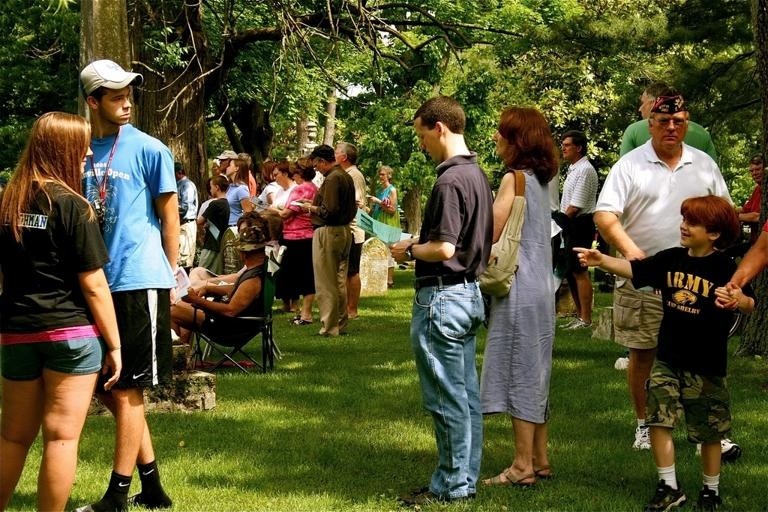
291;315;312;326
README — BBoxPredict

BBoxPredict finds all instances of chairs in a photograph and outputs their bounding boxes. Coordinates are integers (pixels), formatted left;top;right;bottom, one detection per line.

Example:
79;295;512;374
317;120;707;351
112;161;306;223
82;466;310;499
191;259;284;374
200;243;290;365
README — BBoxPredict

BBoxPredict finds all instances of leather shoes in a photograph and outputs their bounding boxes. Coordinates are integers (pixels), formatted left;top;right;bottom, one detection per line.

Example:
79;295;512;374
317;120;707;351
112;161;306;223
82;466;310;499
398;488;475;505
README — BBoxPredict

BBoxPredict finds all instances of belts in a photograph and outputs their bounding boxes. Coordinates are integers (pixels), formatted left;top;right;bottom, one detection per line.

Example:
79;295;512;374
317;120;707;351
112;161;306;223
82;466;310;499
414;275;479;289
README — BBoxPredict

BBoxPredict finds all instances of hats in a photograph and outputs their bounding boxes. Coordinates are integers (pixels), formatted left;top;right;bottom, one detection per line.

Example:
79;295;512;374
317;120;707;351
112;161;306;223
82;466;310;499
650;95;688;114
238;226;265;253
80;59;143;98
216;150;238;161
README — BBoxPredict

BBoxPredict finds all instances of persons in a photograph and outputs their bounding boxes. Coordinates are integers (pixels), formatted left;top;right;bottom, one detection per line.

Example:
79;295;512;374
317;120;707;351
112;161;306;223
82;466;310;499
367;165;403;288
389;97;492;507
172;141;367;345
74;59;179;512
479;105;557;486
0;113;123;512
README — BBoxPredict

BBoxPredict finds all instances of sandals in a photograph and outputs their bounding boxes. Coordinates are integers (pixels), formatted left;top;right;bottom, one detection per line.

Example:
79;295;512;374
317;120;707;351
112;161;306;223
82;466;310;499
482;465;551;488
389;282;395;287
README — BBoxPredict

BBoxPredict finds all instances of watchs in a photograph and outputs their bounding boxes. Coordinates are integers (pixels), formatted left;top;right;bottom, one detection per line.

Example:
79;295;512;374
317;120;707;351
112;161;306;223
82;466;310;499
405;244;413;260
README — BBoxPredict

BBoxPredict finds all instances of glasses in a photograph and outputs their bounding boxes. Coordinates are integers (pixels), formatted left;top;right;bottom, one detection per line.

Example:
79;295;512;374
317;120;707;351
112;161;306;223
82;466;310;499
561;144;576;147
653;117;686;128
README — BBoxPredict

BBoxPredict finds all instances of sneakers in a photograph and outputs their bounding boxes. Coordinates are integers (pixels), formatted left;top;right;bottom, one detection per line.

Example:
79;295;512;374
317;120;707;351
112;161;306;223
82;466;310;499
643;479;687;512
633;426;651;450
697;485;722;510
559;318;592;331
695;438;742;460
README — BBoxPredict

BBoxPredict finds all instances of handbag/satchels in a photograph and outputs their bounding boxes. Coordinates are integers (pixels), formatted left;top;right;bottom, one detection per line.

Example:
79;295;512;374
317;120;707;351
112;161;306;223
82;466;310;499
479;195;525;297
378;208;400;228
